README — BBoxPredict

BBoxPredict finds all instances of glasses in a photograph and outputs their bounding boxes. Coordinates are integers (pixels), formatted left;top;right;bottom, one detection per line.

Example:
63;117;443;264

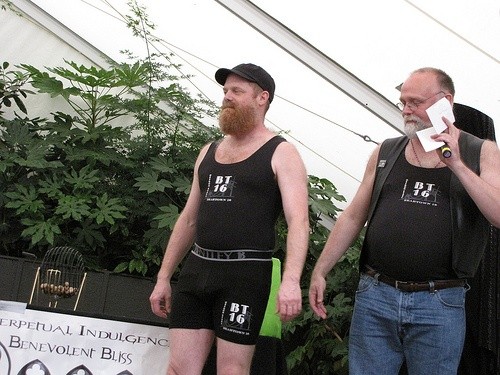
396;91;446;112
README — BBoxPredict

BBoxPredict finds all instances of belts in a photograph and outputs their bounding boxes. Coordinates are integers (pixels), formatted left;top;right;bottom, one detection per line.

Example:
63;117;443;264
365;265;464;293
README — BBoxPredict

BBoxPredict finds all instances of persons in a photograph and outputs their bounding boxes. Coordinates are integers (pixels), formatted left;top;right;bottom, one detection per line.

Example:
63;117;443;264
310;67;500;375
148;63;309;375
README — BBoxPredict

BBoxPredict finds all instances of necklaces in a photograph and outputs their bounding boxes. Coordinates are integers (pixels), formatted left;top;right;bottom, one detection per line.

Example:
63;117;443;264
406;139;442;168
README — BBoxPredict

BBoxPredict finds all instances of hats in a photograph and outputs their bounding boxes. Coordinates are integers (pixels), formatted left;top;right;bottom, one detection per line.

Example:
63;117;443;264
215;63;275;103
